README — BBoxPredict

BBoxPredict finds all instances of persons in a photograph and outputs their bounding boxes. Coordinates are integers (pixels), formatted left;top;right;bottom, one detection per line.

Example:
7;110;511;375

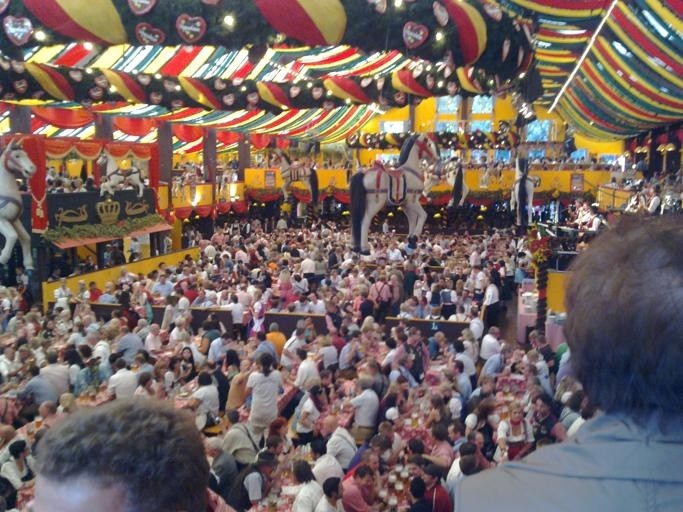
0;130;454;512
438;131;681;241
451;213;682;509
